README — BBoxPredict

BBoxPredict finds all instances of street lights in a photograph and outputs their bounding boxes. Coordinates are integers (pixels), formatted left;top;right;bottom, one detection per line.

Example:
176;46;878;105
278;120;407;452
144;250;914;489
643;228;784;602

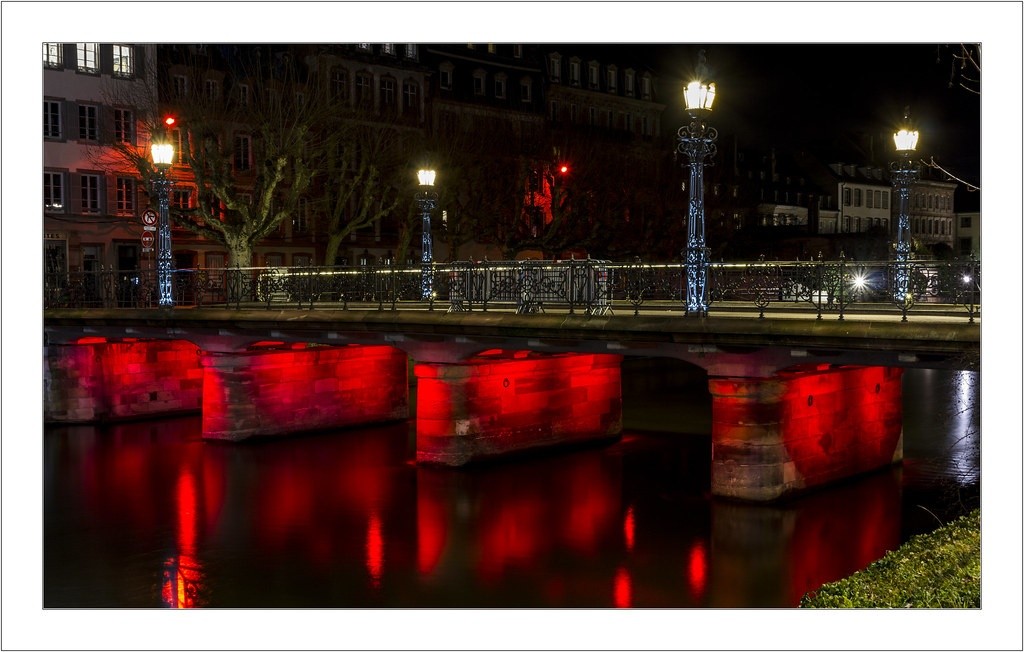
417;156;438;303
151;125;181;305
680;74;719;323
894;105;921;321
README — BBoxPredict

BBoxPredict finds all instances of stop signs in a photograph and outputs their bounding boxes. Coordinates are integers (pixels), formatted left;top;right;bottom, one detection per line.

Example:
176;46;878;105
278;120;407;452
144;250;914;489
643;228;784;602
141;231;154;248
